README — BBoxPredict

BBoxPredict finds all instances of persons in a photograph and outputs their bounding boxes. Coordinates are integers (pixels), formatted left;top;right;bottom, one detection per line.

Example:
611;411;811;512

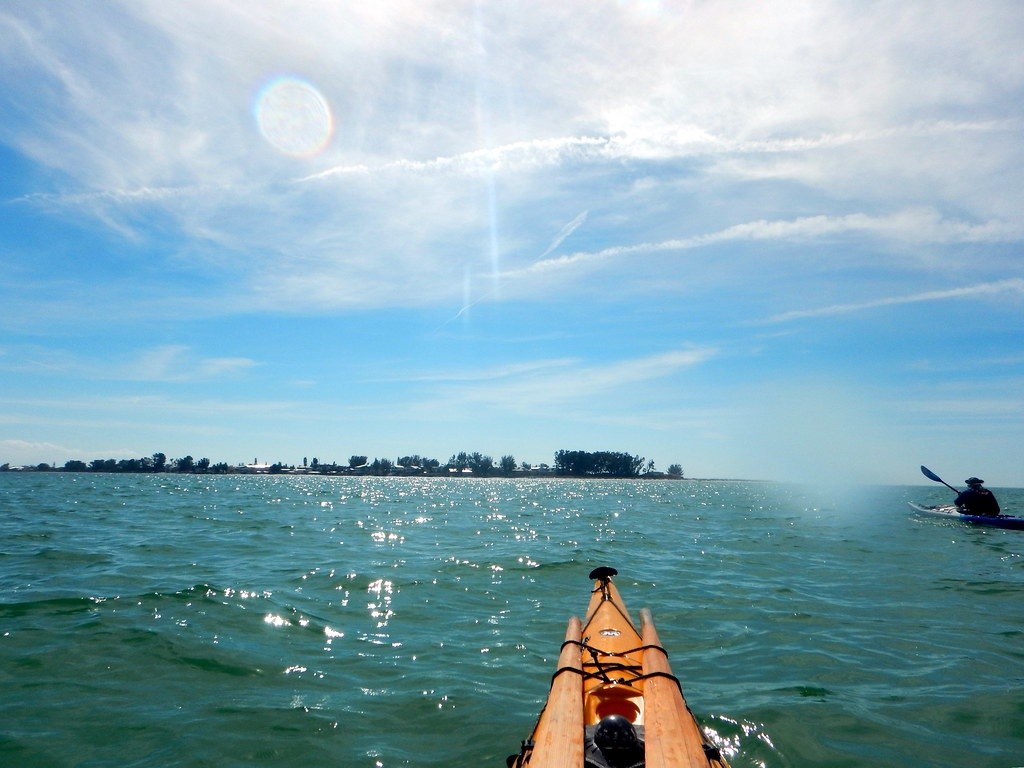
953;476;1000;517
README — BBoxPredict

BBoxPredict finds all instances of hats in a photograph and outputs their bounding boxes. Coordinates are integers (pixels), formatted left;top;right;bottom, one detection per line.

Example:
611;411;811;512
965;477;984;483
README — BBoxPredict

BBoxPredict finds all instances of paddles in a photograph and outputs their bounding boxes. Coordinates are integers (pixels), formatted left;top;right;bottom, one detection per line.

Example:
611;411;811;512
921;465;957;492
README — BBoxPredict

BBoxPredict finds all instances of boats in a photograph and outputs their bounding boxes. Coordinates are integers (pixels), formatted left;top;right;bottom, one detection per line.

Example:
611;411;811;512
908;501;1023;529
